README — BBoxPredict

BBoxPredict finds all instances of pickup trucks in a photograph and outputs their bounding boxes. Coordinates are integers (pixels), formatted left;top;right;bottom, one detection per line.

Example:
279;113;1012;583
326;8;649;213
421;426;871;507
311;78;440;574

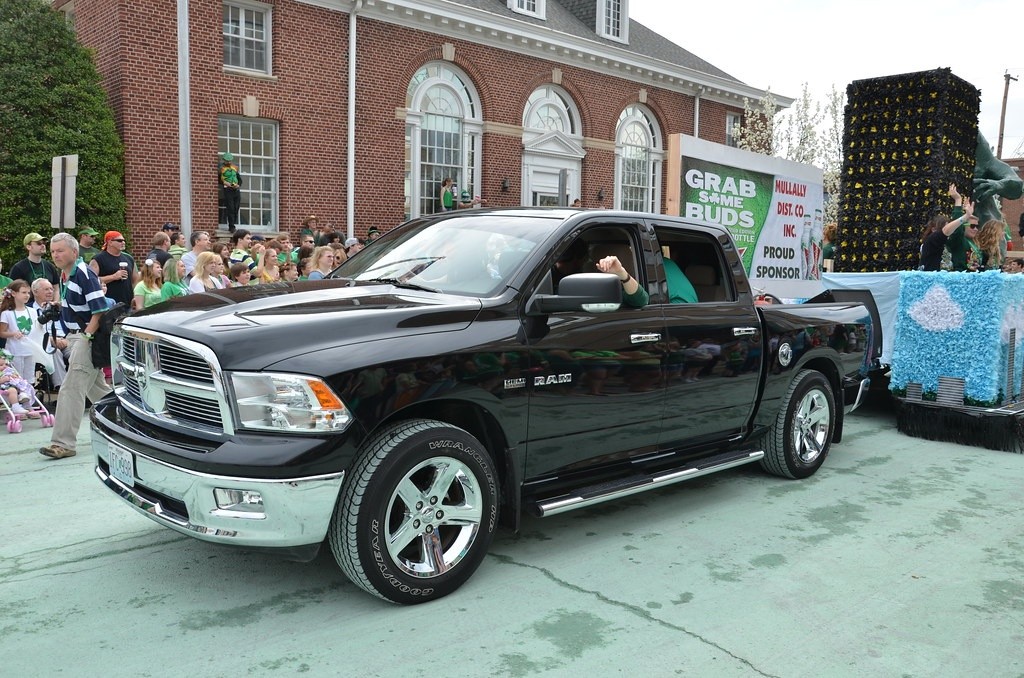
89;208;874;606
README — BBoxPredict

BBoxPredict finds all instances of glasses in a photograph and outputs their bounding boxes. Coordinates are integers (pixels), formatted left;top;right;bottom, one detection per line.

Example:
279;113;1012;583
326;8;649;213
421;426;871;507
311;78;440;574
305;240;316;244
86;235;96;238
280;242;289;244
168;229;178;232
111;239;125;243
964;224;980;230
28;241;46;246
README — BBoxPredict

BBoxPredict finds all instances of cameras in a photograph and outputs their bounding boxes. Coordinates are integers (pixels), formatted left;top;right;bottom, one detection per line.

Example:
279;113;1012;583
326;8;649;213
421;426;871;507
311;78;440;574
37;302;61;325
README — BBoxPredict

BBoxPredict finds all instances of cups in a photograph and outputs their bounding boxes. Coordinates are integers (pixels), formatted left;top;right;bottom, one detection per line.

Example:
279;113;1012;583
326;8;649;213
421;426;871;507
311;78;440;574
120;262;128;279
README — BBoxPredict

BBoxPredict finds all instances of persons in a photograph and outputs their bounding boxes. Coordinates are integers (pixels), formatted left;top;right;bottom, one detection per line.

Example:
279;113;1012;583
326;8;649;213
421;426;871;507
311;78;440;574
657;237;698;303
0;213;380;420
39;233;113;458
458;192;487;210
334;324;867;430
822;224;838;273
971;131;1024;268
571;199;605;209
552;237;649;310
440;178;453;212
218;153;242;233
920;183;1024;273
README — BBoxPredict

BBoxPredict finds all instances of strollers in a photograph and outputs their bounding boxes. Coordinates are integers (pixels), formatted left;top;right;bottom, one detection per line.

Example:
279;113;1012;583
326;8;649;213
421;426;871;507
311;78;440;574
0;349;55;433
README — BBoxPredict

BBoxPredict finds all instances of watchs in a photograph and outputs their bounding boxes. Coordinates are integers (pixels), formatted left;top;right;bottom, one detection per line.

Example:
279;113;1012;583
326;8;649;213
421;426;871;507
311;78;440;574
83;332;94;338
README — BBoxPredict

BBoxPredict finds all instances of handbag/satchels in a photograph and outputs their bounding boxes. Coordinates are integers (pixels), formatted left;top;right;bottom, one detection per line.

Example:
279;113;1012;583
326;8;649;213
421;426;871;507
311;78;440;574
91;301;127;368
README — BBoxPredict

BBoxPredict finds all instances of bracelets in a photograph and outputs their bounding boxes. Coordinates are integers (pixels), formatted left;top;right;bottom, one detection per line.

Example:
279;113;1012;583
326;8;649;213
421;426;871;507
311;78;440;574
959;217;963;224
620;274;631;283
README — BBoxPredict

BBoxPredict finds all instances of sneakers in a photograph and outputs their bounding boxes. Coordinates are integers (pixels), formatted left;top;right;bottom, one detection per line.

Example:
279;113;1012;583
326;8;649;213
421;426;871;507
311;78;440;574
39;446;76;458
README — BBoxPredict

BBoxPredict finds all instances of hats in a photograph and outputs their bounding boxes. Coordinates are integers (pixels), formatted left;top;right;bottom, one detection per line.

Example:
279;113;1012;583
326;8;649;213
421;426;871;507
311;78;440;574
344;238;358;249
163;223;179;229
23;233;48;245
223;153;233;161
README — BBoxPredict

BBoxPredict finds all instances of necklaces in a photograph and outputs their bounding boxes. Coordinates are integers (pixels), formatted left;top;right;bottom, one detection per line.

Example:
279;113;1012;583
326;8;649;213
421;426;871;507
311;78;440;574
176;282;189;293
14;305;32;335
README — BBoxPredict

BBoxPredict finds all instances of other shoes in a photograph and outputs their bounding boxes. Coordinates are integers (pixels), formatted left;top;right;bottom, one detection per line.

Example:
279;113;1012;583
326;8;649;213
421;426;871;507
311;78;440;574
18;394;30;403
13;408;29;414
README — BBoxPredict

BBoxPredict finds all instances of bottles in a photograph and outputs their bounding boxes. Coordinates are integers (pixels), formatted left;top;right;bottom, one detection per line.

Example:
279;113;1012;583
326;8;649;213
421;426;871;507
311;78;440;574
801;215;814;279
1006;240;1013;251
807;209;824;281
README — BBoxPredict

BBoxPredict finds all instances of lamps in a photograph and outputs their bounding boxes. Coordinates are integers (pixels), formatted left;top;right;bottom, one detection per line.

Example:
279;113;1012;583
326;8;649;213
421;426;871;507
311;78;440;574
597;189;604;201
502;179;510;192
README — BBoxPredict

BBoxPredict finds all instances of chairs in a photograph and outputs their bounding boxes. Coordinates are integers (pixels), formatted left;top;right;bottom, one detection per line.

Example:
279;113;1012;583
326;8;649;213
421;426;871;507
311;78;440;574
498;249;554;296
556;237;727;302
